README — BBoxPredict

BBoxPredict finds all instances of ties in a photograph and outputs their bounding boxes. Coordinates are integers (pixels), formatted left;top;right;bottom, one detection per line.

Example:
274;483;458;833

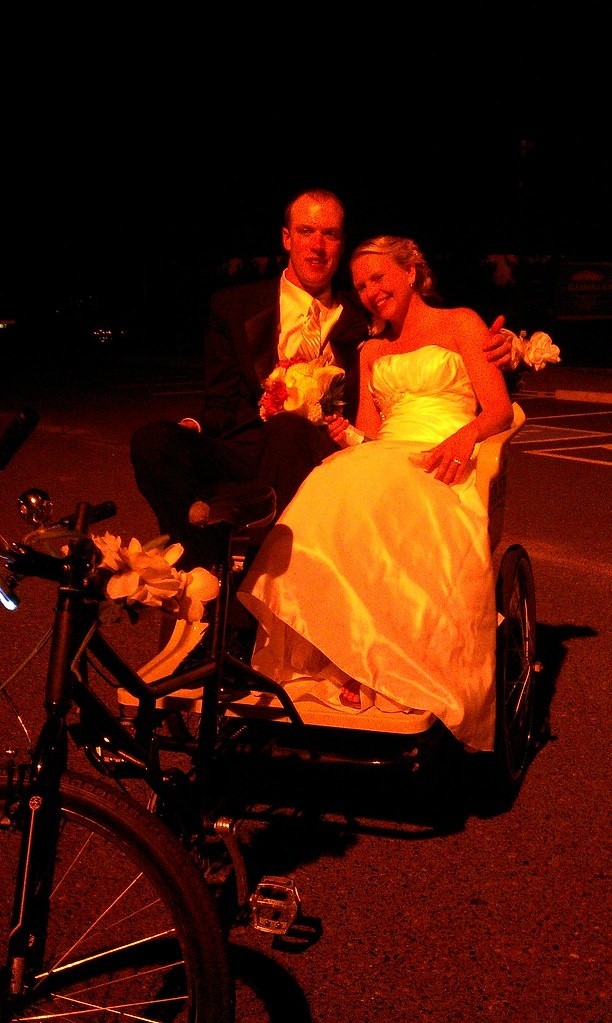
289;298;321;364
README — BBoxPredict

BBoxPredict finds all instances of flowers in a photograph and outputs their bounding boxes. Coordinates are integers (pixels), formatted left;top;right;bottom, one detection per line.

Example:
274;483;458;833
89;530;220;623
257;352;365;447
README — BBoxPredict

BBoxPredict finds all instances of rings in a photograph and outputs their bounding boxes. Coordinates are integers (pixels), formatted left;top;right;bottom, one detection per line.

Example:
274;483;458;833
454;459;462;465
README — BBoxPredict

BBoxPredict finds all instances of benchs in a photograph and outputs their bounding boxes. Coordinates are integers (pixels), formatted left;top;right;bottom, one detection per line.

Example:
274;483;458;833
475;402;527;555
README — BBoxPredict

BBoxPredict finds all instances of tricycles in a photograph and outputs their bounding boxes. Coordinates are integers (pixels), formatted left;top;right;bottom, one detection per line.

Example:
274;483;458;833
0;398;545;1023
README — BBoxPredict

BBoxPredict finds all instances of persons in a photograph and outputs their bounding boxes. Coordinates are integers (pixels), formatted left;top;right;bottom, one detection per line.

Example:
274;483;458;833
129;187;530;696
236;237;513;752
